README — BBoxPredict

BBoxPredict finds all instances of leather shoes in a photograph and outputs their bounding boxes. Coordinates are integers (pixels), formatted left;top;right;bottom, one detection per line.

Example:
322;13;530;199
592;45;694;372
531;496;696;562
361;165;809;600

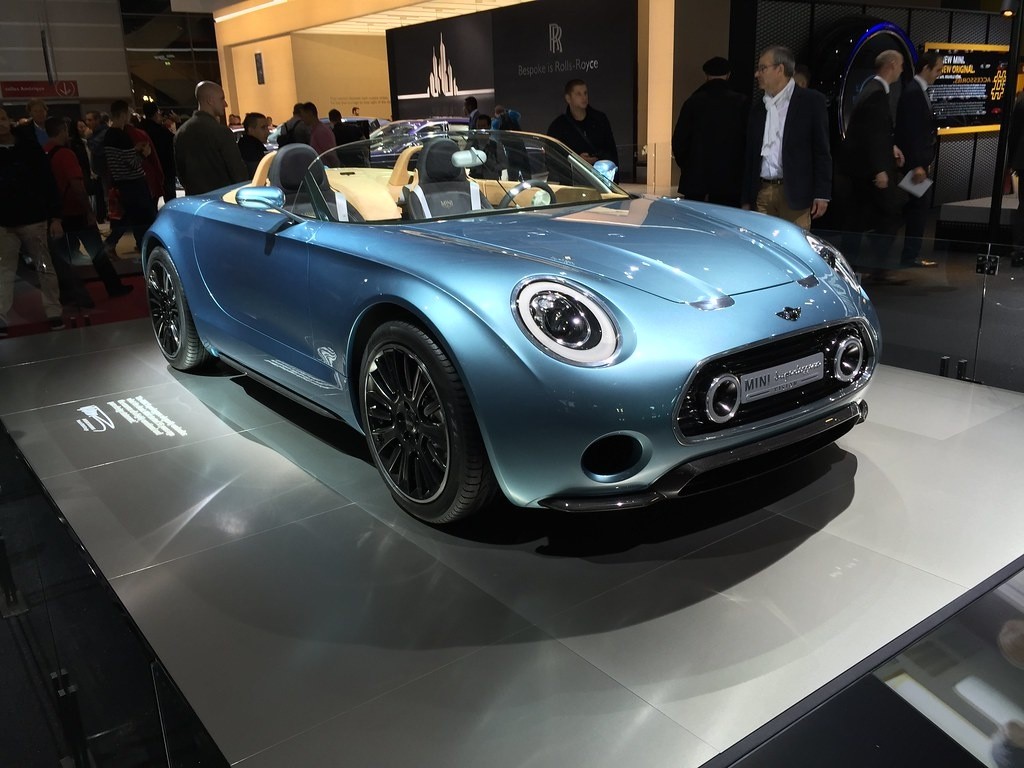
901;258;940;268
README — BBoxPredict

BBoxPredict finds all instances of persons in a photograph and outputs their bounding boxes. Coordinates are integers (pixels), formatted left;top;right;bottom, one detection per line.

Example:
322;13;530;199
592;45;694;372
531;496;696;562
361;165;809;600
544;79;619;185
281;102;367;167
1007;90;1024;266
463;97;532;181
0;97;181;329
173;81;277;195
671;45;942;285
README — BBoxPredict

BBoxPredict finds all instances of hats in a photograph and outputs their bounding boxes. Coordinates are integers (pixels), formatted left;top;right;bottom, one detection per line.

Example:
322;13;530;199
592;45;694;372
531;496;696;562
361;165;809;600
703;56;730;76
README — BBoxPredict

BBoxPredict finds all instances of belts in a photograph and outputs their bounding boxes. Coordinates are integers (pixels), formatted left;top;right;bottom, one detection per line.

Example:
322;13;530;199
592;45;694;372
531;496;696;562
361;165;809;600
760;177;784;184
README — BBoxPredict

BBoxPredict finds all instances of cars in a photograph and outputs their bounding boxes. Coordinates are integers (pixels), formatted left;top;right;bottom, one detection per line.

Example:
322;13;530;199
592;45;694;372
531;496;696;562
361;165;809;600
231;116;549;183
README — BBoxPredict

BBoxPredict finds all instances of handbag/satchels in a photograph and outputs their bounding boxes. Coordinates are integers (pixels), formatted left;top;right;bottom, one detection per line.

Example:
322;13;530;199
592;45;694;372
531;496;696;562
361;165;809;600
108;188;122;220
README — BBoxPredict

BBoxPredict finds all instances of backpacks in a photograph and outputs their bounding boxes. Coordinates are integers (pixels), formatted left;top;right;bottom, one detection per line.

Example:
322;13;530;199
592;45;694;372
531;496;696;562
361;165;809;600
277;120;301;149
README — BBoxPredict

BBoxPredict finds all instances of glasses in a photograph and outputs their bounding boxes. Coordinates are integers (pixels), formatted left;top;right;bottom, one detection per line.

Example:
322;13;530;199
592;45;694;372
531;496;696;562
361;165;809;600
754;63;779;73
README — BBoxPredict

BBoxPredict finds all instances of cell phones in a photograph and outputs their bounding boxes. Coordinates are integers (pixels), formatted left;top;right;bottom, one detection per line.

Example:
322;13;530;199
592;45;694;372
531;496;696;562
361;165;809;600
589;153;597;157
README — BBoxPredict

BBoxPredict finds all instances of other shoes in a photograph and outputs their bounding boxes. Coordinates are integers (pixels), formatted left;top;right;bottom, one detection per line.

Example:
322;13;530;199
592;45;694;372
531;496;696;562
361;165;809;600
104;244;120;261
0;327;9;336
1011;254;1024;267
49;316;65;330
110;284;134;297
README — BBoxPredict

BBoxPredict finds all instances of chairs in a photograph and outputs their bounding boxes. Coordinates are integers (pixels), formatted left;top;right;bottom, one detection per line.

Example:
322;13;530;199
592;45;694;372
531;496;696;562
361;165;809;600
404;137;494;219
270;142;366;222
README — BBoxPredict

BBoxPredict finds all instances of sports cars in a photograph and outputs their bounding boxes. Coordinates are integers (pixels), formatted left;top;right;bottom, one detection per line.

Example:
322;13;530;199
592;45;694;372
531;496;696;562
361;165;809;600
142;128;880;527
